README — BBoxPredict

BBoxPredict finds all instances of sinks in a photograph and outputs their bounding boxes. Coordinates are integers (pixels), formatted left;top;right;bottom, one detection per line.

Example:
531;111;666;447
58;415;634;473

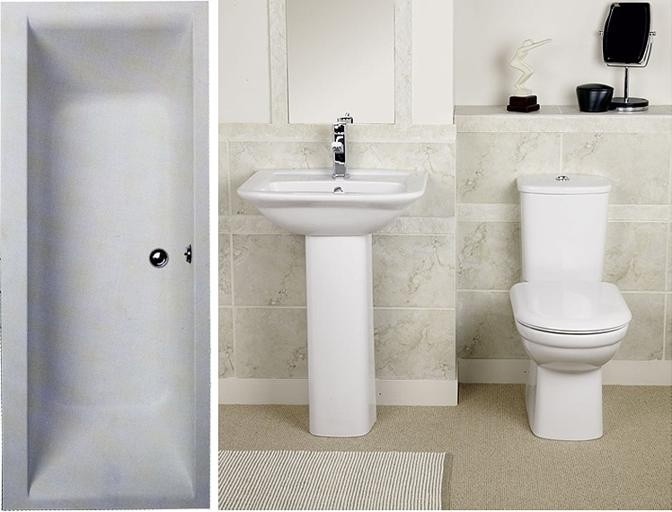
237;168;430;236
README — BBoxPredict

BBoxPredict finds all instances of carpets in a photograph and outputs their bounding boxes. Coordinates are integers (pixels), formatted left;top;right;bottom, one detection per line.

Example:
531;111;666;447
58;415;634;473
218;449;452;511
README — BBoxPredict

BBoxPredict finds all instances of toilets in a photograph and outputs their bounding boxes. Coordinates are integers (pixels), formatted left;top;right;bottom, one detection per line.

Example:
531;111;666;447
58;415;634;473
511;175;634;441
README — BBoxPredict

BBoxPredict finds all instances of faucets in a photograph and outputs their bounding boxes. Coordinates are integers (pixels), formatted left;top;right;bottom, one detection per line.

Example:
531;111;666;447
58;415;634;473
331;112;354;180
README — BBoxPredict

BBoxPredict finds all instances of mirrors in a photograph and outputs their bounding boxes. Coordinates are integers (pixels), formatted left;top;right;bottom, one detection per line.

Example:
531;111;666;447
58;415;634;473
599;2;656;112
